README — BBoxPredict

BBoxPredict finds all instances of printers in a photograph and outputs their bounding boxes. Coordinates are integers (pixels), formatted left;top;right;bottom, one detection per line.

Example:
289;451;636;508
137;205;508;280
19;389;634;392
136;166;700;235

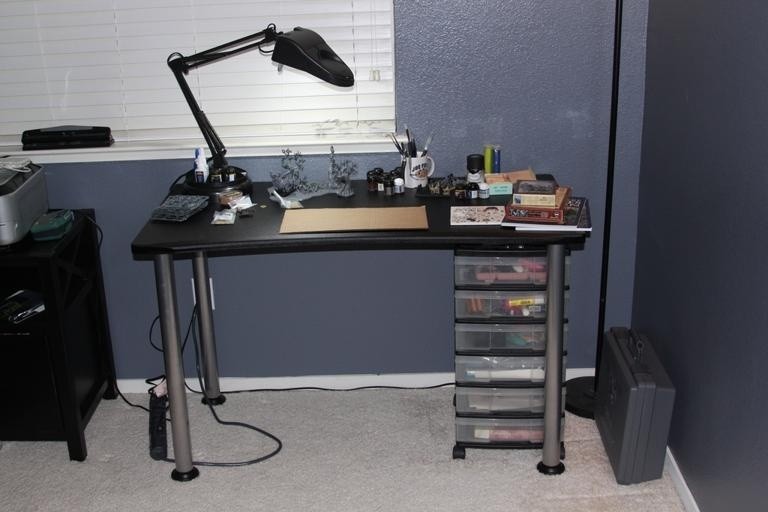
0;160;49;247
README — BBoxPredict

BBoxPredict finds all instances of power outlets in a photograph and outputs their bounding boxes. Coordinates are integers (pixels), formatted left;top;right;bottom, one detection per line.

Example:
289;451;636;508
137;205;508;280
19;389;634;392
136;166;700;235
191;278;216;312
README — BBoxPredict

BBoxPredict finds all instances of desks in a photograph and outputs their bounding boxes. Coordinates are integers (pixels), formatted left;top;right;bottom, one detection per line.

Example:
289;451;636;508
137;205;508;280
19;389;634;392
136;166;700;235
131;175;591;481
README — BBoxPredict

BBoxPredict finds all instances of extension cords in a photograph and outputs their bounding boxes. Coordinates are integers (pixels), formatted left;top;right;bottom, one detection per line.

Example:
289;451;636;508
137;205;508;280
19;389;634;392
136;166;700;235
149;389;168;460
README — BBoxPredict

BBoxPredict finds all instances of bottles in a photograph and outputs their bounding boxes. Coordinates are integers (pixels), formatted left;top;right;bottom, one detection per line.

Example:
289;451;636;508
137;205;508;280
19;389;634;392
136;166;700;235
454;181;490;200
367;166;405;196
195;148;210;183
465;153;485;182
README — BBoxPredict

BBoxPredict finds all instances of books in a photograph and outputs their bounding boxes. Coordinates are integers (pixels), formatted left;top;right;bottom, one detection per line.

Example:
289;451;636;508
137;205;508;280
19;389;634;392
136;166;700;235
501;179;593;232
450;206;506;226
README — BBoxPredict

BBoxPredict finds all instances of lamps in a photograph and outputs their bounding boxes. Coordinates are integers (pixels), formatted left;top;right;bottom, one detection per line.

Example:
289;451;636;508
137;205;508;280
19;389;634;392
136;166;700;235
168;24;354;209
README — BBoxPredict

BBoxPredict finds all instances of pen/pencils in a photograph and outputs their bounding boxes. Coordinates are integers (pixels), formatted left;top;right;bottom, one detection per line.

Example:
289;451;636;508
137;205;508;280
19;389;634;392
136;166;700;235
386;124;435;156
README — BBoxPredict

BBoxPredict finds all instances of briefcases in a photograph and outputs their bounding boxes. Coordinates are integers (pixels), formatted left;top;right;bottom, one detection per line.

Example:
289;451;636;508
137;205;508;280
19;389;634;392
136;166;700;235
593;324;676;486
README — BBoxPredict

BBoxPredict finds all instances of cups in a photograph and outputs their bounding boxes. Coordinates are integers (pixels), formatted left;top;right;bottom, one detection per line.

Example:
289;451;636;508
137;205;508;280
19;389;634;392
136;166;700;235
400;151;435;188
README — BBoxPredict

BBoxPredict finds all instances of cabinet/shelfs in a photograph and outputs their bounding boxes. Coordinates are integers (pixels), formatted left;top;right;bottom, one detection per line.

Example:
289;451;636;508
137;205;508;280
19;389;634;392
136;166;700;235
0;209;118;463
453;249;572;458
